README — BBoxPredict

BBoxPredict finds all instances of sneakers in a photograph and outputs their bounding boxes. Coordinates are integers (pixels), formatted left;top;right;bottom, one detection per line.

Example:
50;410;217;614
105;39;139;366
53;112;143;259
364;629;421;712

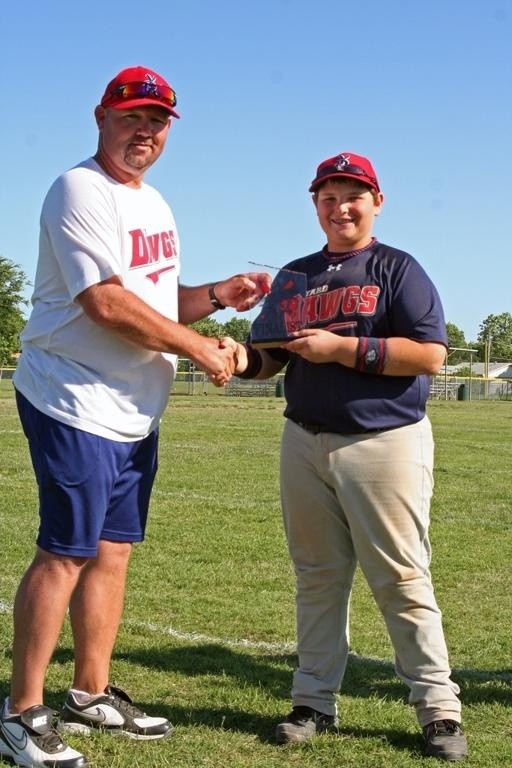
275;706;344;743
1;696;84;768
61;689;172;741
424;720;467;760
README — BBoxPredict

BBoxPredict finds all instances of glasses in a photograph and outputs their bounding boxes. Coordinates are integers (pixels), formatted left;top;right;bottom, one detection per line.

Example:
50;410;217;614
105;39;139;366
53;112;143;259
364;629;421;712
318;164;376;183
101;82;177;107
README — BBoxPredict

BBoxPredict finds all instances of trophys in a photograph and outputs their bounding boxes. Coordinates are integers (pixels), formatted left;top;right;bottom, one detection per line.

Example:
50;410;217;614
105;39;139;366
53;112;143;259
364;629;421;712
247;261;307;350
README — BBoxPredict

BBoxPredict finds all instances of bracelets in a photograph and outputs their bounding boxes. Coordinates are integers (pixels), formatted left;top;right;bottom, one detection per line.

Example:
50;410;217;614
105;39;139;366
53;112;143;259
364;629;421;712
235;342;261;381
356;337;387;376
210;281;226;309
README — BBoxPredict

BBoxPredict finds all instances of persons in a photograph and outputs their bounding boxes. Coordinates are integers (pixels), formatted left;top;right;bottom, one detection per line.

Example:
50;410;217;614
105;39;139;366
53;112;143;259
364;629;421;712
0;66;271;768
209;152;470;763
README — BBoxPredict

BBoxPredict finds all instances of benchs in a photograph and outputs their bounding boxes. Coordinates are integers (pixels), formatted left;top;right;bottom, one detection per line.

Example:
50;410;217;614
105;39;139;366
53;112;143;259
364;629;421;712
224;378;270;397
429;382;462;401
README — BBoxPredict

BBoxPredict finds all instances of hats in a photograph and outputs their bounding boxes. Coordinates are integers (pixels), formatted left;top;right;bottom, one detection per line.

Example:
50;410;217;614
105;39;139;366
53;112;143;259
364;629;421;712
309;152;379;191
101;66;181;118
